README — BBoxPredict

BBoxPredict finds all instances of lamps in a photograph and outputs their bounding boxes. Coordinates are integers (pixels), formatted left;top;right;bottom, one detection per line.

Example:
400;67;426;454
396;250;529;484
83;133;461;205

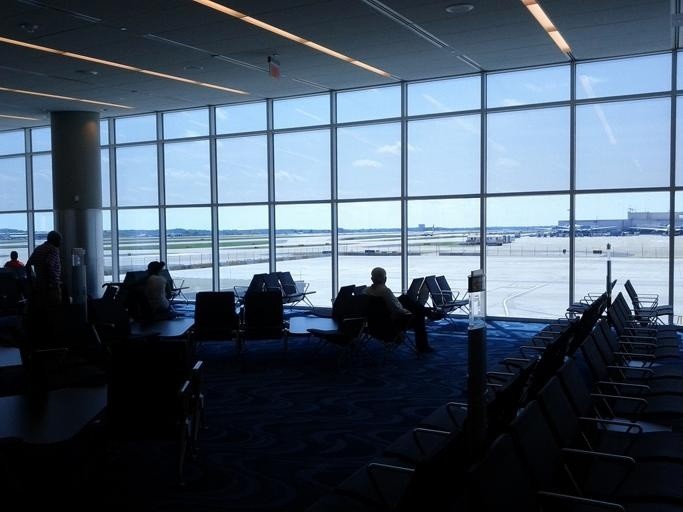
100;268;187;313
192;270;315;369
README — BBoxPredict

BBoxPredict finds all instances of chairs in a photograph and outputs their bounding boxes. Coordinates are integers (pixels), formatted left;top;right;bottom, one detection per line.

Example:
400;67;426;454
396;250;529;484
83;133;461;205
100;268;187;313
369;281;682;512
310;274;473;364
192;270;315;369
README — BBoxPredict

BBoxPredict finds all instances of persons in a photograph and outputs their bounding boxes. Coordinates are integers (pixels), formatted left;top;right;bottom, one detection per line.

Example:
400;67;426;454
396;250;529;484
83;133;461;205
159;262;173;290
361;268;434;354
25;231;62;302
141;262;177;320
4;251;24;268
